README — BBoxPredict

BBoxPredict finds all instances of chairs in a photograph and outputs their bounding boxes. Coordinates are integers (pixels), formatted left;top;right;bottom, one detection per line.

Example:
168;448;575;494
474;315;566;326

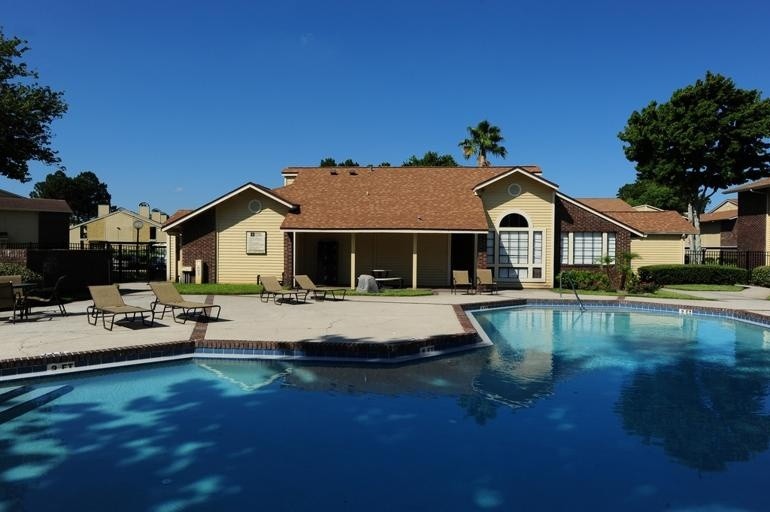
0;274;69;325
451;269;498;295
258;274;347;305
87;281;221;331
